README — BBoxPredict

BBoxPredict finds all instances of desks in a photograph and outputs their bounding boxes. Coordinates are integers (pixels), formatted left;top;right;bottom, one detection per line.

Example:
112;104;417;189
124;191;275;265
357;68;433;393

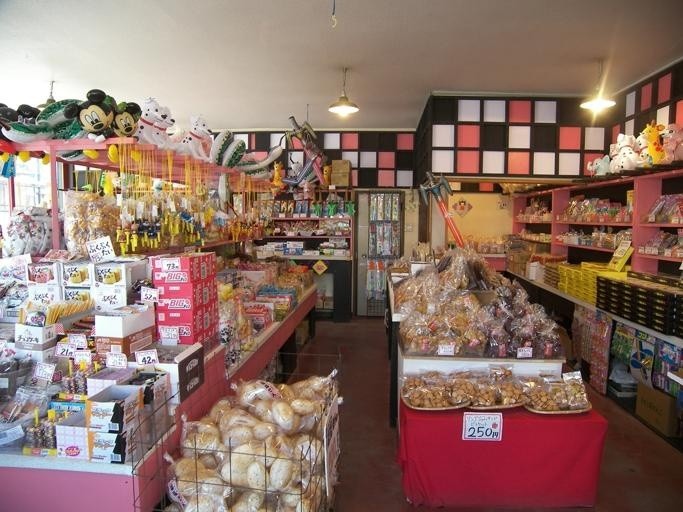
397;389;609;511
384;270;408;429
394;329;567;438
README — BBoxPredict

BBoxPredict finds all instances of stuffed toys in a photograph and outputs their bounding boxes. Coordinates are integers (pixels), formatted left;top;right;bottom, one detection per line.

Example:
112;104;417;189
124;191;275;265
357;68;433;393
0;90;286;190
587;119;683;176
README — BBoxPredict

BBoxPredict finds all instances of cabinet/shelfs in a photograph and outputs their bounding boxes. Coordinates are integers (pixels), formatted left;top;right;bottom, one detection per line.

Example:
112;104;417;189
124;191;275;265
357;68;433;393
0;133;353;511
510;168;683;454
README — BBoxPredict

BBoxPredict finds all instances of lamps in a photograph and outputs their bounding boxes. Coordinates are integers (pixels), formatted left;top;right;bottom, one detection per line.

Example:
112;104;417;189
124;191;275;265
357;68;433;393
326;67;358;118
577;59;617;110
33;81;57;109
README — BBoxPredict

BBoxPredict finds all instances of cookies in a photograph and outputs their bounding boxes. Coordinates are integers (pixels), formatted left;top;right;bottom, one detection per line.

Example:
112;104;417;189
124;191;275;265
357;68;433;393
405;368;589;411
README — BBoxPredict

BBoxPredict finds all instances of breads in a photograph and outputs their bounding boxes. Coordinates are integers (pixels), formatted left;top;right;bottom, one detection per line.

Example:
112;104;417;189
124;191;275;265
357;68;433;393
164;375;330;512
